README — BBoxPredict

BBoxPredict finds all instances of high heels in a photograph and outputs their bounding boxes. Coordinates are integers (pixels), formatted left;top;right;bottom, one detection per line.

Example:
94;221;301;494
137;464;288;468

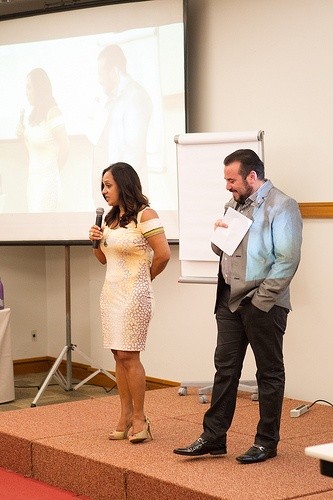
107;422;132;439
131;417;153;442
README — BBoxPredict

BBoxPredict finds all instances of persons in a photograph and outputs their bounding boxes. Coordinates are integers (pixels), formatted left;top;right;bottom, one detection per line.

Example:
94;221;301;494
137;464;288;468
16;65;66;210
93;45;164;182
174;149;303;464
88;162;171;442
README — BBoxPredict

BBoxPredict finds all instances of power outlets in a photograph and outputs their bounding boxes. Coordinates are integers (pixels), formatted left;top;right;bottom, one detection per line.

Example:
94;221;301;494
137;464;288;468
31;329;37;343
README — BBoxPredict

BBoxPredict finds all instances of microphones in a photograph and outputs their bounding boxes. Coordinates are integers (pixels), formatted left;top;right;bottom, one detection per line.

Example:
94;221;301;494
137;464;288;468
224;203;234;216
93;207;104;249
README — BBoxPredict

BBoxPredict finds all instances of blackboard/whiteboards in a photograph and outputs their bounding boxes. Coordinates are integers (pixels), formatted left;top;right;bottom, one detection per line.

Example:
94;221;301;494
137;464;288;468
172;128;265;285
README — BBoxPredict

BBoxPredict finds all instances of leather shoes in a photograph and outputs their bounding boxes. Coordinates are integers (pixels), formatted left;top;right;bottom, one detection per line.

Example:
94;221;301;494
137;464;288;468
173;438;228;456
235;443;278;463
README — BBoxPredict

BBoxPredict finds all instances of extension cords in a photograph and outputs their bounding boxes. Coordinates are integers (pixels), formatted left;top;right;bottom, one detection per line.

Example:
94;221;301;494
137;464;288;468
290;405;310;417
